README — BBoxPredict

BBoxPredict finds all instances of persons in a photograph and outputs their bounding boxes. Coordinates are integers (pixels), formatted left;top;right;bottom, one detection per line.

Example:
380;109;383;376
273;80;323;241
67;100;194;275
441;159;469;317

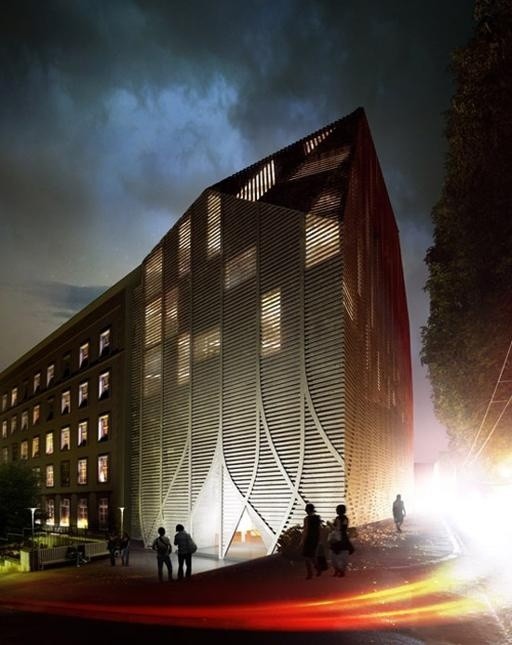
66;539;87;569
108;527;130;567
152;527;173;584
327;505;354;577
297;504;322;580
392;495;404;532
174;523;197;582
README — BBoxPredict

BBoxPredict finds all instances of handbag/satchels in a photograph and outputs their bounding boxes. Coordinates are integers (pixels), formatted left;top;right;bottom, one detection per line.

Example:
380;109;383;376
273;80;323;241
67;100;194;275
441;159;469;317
168;544;172;555
185;533;197;554
326;518;342;545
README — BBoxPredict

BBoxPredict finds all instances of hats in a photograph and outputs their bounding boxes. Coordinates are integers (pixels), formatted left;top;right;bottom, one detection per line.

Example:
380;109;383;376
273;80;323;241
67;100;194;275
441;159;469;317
304;503;317;512
